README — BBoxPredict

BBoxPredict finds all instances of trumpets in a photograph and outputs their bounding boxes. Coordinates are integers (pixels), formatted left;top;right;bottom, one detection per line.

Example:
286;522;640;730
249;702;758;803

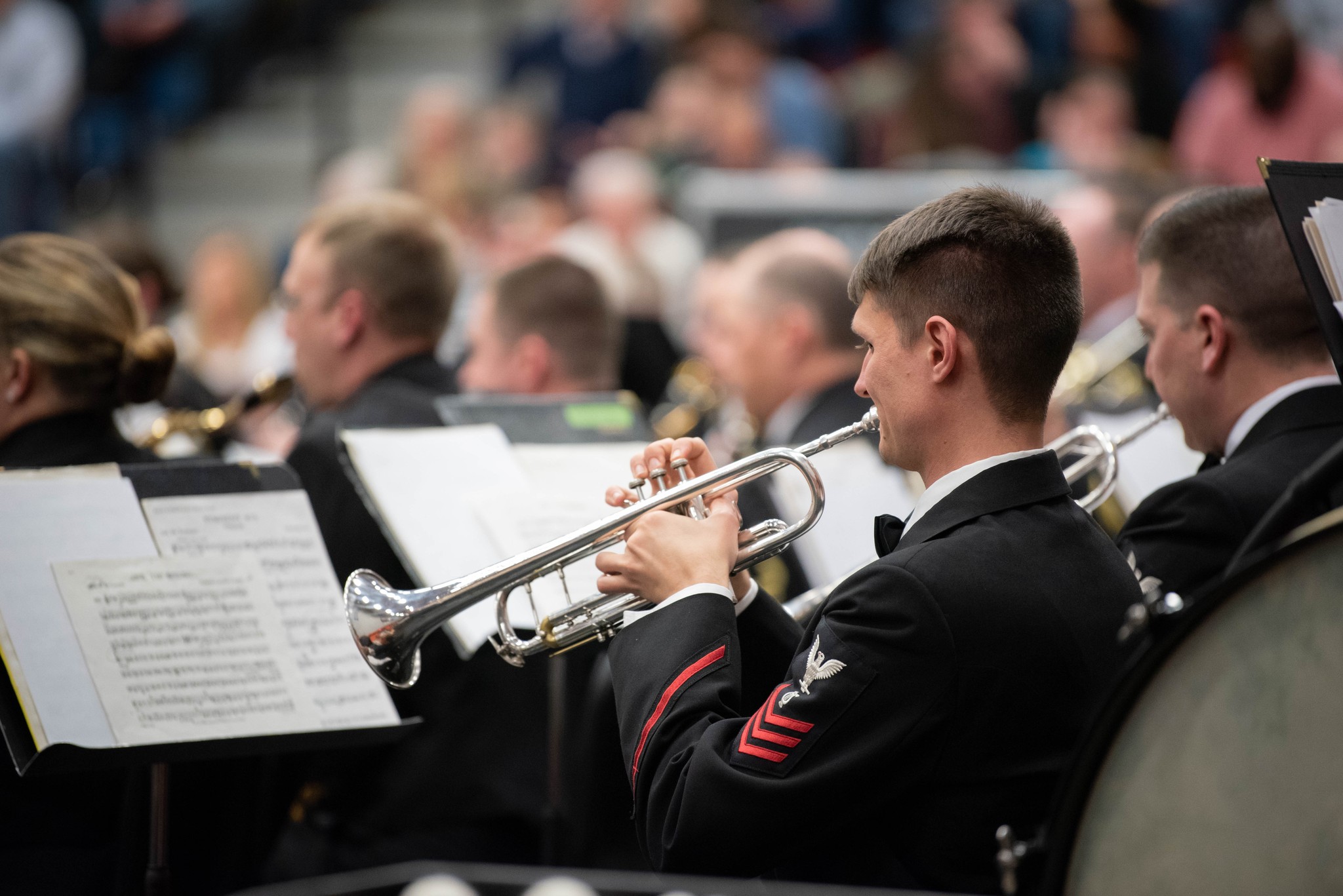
340;403;886;690
782;400;1175;633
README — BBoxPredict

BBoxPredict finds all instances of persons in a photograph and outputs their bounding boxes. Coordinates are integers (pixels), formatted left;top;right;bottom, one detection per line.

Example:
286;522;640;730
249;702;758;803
590;182;1143;896
1083;184;1343;681
0;0;1209;454
